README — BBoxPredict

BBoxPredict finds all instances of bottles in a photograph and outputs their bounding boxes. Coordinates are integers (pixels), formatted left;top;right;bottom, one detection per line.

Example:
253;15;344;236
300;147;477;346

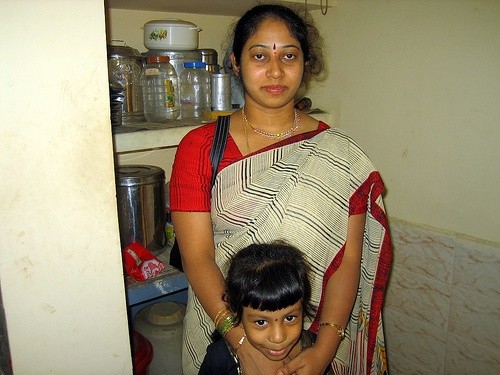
178;62;212;127
143;55;179;130
199;48;224;74
107;40;145;132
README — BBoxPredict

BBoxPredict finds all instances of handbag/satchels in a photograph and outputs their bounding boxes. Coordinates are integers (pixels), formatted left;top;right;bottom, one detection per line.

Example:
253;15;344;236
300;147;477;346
170;114;232;273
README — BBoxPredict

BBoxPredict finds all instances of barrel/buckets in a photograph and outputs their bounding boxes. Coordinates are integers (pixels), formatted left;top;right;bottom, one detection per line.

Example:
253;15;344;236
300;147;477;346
114;164;172;258
146;50;202;91
129;324;153;375
133;301;187;375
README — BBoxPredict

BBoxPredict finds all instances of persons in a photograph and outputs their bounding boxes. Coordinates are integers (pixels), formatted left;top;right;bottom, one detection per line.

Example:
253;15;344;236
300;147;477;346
198;240;335;375
168;5;393;375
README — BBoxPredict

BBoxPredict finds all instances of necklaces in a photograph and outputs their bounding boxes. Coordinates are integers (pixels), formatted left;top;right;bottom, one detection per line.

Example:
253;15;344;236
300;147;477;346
242;105;300;154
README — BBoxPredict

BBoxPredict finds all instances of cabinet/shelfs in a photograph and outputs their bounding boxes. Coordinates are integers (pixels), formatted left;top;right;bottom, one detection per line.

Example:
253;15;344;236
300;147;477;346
113;114;333;306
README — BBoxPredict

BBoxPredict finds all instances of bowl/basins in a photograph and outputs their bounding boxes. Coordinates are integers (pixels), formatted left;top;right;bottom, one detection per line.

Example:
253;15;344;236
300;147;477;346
144;19;202;50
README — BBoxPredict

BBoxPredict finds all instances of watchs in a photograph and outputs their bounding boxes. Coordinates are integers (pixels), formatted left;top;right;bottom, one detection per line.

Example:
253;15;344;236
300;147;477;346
318;322;345;340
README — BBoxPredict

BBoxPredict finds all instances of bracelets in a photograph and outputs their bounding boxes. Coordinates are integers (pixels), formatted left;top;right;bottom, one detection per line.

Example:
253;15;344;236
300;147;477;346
233;332;248;354
214;308;240;339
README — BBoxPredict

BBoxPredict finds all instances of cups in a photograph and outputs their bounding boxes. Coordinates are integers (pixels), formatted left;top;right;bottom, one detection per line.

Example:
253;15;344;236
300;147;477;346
211;73;232;112
